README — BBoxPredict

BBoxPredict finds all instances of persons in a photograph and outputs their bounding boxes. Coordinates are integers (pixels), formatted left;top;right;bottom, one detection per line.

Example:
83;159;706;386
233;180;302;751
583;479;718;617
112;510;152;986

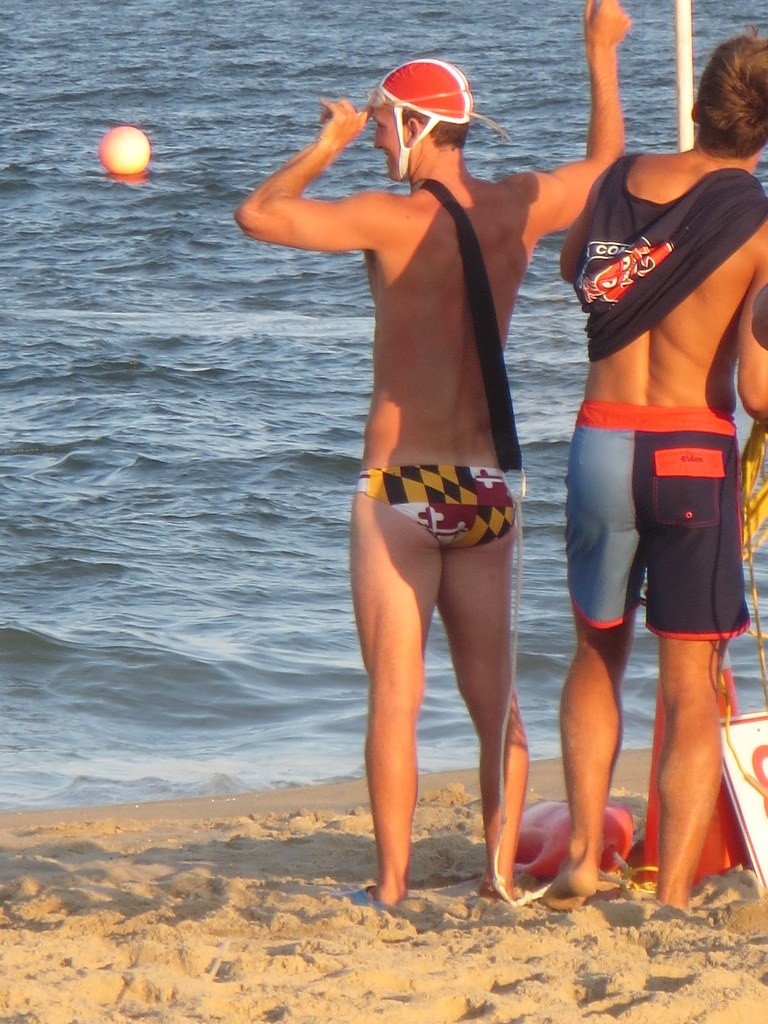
232;0;630;916
548;33;768;917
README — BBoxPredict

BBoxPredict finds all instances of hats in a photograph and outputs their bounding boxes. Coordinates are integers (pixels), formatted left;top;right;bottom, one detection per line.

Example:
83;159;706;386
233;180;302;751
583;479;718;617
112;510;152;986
365;58;474;124
